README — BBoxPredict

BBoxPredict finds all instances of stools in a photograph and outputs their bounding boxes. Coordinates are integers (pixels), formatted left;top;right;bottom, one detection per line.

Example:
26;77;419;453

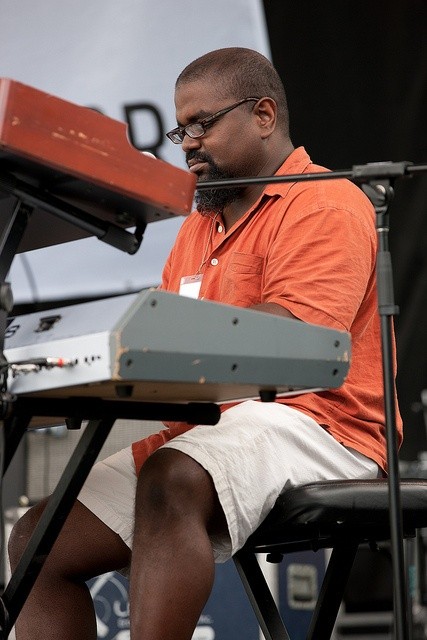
229;476;425;640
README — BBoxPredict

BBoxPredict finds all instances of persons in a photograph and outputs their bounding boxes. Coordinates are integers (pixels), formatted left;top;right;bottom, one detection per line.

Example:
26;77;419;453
8;46;404;640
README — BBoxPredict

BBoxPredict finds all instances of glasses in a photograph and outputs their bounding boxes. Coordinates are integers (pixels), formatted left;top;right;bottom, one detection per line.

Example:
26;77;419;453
166;96;260;145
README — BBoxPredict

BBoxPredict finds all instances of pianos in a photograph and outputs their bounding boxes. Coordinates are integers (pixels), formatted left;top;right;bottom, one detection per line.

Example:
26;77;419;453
5;288;352;404
